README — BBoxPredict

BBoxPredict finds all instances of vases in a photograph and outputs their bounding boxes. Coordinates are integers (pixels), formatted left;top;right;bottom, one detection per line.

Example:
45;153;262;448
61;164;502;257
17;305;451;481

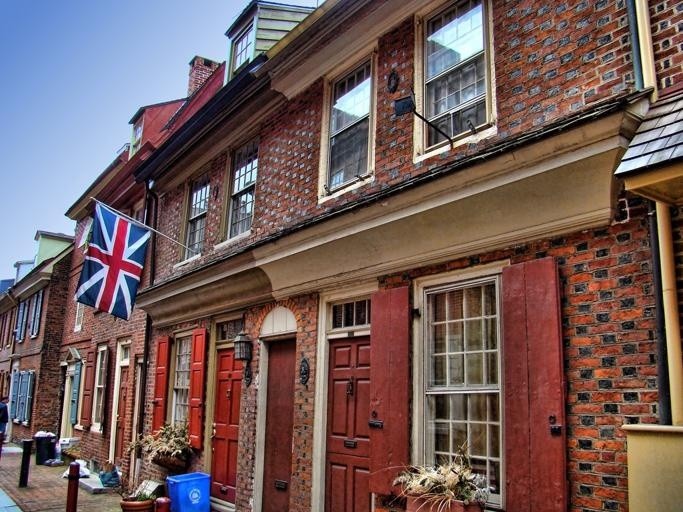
407;493;483;512
151;453;190;472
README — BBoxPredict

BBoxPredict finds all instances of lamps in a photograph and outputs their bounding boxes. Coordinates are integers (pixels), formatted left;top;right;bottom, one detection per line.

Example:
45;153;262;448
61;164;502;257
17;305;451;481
395;96;453;150
233;327;253;360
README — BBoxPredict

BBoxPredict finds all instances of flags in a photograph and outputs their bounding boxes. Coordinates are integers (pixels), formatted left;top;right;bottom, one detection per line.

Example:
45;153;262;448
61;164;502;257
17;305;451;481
72;201;153;321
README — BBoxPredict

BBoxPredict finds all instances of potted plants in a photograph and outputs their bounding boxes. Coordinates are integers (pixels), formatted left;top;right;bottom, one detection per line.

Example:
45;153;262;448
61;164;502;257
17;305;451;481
120;490;154;512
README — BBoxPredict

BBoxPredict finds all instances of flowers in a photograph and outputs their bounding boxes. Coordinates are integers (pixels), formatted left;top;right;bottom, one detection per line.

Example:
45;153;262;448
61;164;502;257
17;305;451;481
127;424;191;458
391;440;487;512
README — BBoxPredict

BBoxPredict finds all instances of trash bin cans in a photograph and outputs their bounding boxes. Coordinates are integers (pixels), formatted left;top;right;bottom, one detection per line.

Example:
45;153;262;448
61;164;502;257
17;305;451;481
166;472;211;512
32;434;57;466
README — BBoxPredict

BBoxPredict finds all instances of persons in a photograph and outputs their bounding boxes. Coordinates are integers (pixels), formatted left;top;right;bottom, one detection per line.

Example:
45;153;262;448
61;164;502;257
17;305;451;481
0;396;9;444
